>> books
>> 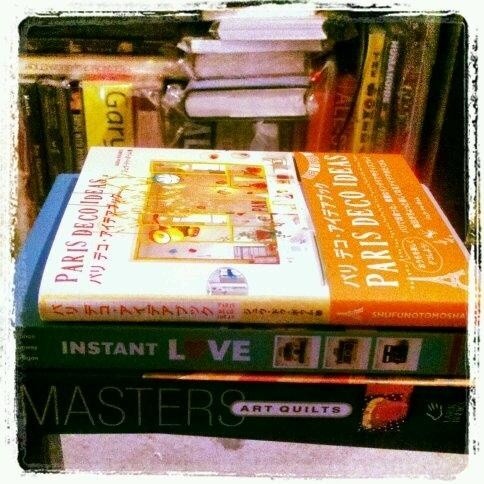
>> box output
[17,11,468,252]
[16,145,472,382]
